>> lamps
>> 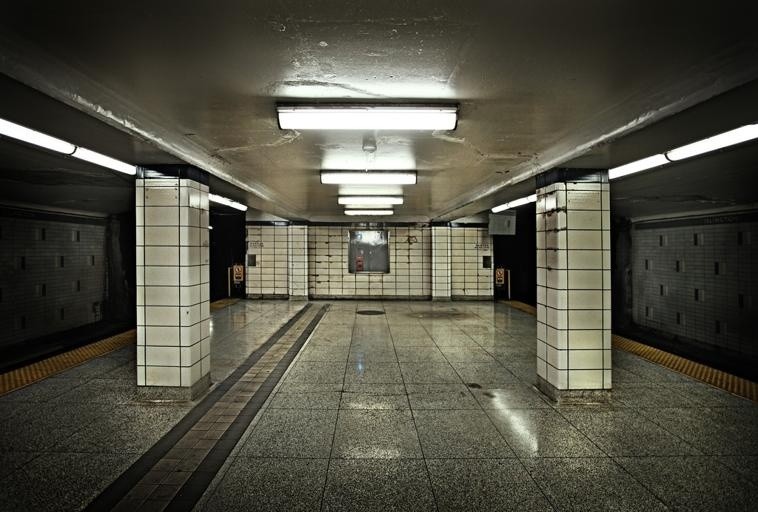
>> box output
[491,124,758,213]
[275,102,459,216]
[0,119,248,211]
